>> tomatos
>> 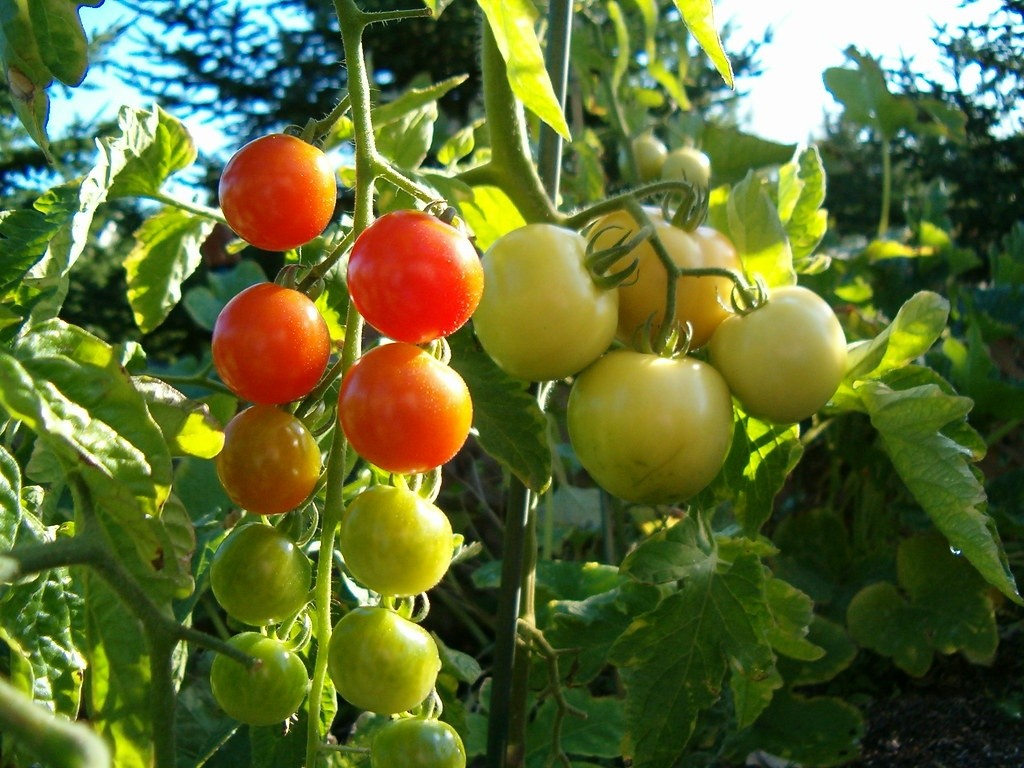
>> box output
[207,132,848,767]
